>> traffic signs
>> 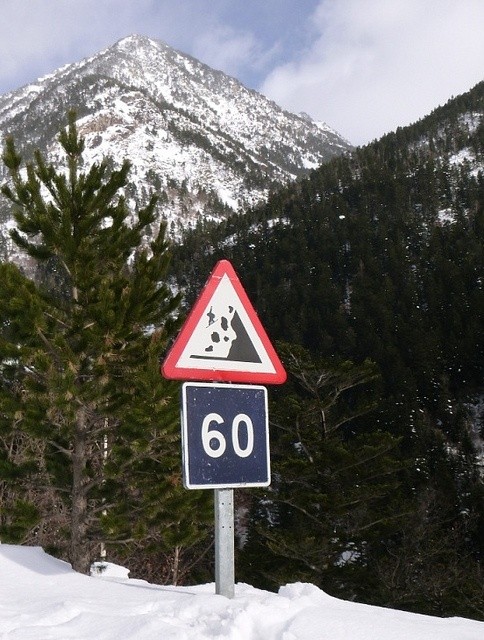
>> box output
[182,381,274,490]
